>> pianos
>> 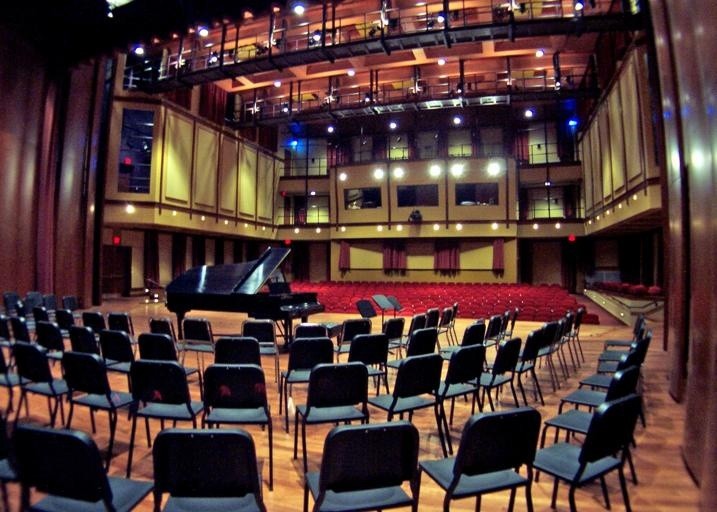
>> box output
[165,246,325,341]
